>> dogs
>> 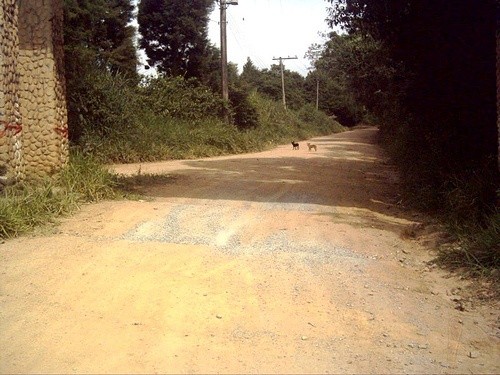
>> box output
[307,143,317,151]
[291,141,299,149]
